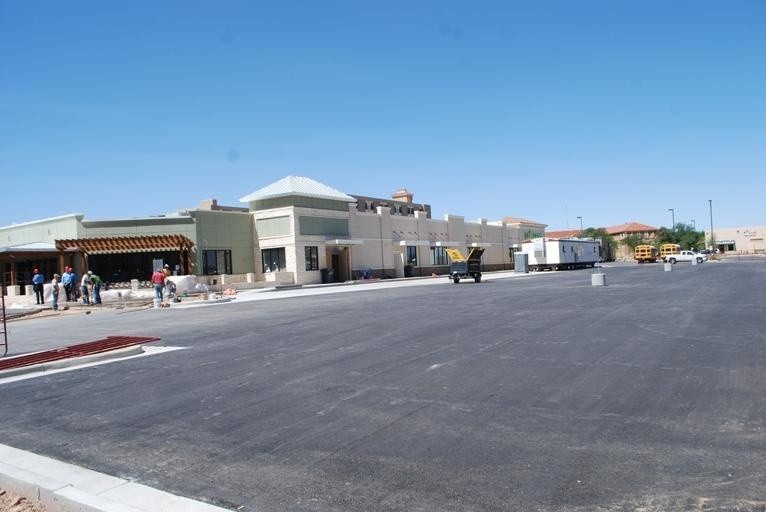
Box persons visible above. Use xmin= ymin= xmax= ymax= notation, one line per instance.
xmin=81 ymin=270 xmax=93 ymax=304
xmin=32 ymin=269 xmax=44 ymax=305
xmin=152 ymin=269 xmax=164 ymax=302
xmin=67 ymin=268 xmax=78 ymax=302
xmin=88 ymin=270 xmax=103 ymax=304
xmin=162 ymin=264 xmax=170 ymax=280
xmin=61 ymin=266 xmax=72 ymax=302
xmin=166 ymin=280 xmax=176 ymax=298
xmin=52 ymin=274 xmax=61 ymax=311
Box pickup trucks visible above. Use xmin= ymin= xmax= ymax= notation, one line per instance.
xmin=664 ymin=250 xmax=708 ymax=264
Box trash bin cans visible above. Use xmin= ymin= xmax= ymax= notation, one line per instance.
xmin=320 ymin=268 xmax=336 ymax=284
xmin=404 ymin=263 xmax=415 ymax=278
